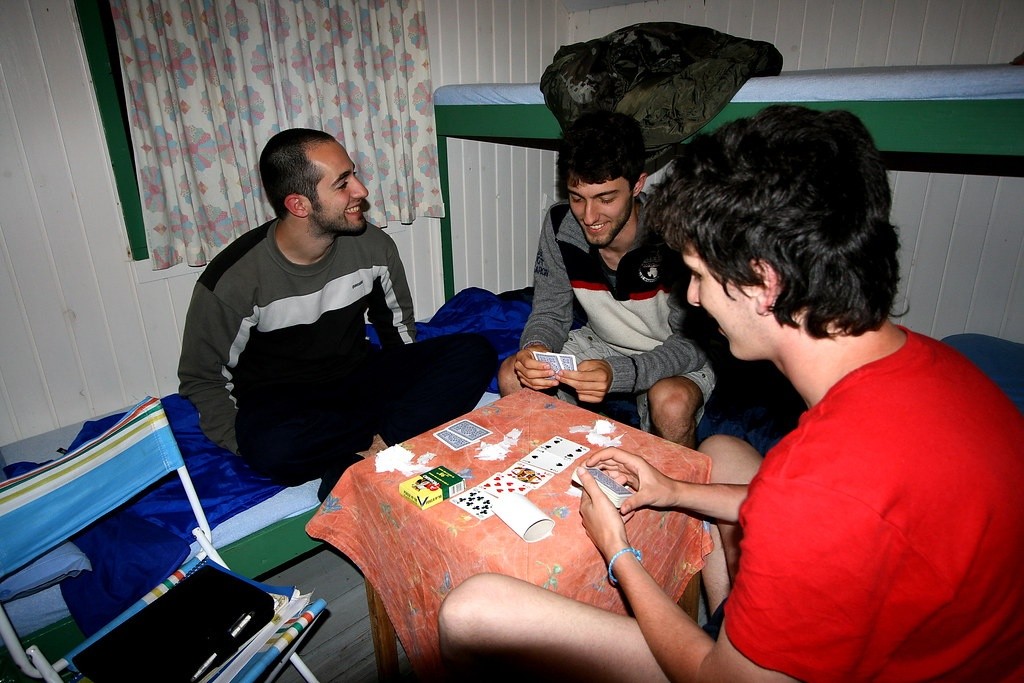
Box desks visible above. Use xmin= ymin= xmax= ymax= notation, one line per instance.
xmin=305 ymin=388 xmax=715 ymax=683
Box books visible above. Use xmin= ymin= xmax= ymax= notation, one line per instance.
xmin=71 ymin=552 xmax=300 ymax=683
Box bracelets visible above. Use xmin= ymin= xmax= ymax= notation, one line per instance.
xmin=607 ymin=548 xmax=642 ymax=583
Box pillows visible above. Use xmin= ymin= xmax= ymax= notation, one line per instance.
xmin=940 ymin=333 xmax=1024 ymax=416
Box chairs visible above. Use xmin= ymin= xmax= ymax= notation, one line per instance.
xmin=0 ymin=396 xmax=327 ymax=683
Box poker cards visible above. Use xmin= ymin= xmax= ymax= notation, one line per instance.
xmin=566 ymin=465 xmax=635 ymax=509
xmin=448 ymin=435 xmax=590 ymax=521
xmin=533 ymin=349 xmax=578 ymax=379
xmin=433 ymin=418 xmax=494 ymax=452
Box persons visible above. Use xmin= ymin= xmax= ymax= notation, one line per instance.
xmin=436 ymin=103 xmax=1024 ymax=682
xmin=496 ymin=106 xmax=721 ymax=454
xmin=179 ymin=128 xmax=498 ymax=506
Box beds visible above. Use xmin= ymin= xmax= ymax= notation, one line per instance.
xmin=0 ymin=315 xmax=500 ymax=683
xmin=433 ymin=64 xmax=1024 ymax=459
